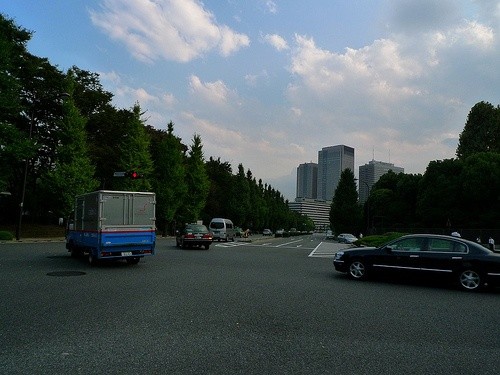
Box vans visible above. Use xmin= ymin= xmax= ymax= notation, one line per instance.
xmin=210 ymin=218 xmax=237 ymax=243
xmin=326 ymin=230 xmax=335 ymax=239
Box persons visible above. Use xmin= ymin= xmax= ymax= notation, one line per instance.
xmin=488 ymin=235 xmax=495 ymax=253
xmin=360 ymin=232 xmax=363 ymax=238
xmin=476 ymin=236 xmax=482 ymax=245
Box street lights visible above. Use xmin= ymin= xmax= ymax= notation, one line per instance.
xmin=16 ymin=91 xmax=71 ymax=240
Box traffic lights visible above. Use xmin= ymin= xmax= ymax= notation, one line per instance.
xmin=128 ymin=170 xmax=145 ymax=179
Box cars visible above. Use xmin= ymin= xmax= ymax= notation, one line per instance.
xmin=175 ymin=223 xmax=213 ymax=250
xmin=263 ymin=229 xmax=273 ymax=236
xmin=332 ymin=233 xmax=500 ymax=293
xmin=276 ymin=228 xmax=314 ymax=234
xmin=337 ymin=234 xmax=358 ymax=244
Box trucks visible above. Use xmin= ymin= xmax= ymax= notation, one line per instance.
xmin=64 ymin=191 xmax=157 ymax=267
xmin=290 ymin=228 xmax=296 ymax=233
